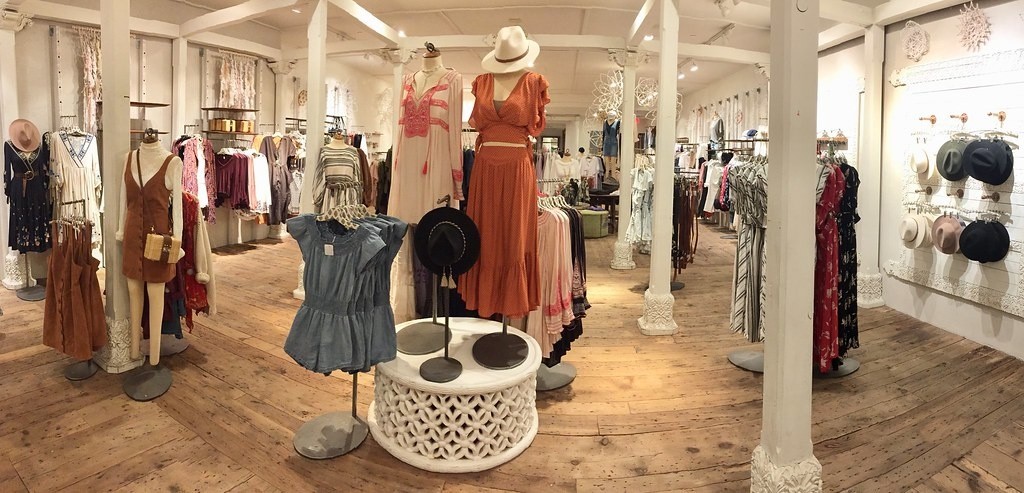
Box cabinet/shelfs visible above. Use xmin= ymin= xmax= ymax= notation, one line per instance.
xmin=202 ymin=107 xmax=260 ymax=142
xmin=95 ymin=99 xmax=170 ymax=136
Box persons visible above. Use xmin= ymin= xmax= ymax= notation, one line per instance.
xmin=600 ymin=110 xmax=622 ymax=180
xmin=3 ymin=119 xmax=53 ymax=301
xmin=310 ymin=131 xmax=366 ymax=218
xmin=115 ymin=127 xmax=184 ymax=367
xmin=450 ymin=24 xmax=550 ymax=372
xmin=705 ymin=110 xmax=725 ymax=145
xmin=385 ymin=41 xmax=465 ymax=357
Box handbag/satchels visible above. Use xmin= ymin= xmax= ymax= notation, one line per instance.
xmin=144 ymin=233 xmax=181 ymax=265
xmin=210 ymin=117 xmax=255 ymax=133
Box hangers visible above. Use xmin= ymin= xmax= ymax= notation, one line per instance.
xmin=4 ymin=116 xmax=1020 ymax=249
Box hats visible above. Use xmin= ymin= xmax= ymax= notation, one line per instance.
xmin=896 ymin=213 xmax=1010 ymax=264
xmin=963 ymin=138 xmax=1014 ymax=190
xmin=481 ymin=25 xmax=541 ymax=74
xmin=412 ymin=207 xmax=481 ymax=288
xmin=905 ymin=143 xmax=934 ymax=181
xmin=8 ymin=118 xmax=41 ymax=152
xmin=936 ymin=139 xmax=968 ymax=181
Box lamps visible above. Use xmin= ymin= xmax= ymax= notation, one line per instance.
xmin=733 ymin=0 xmax=741 ymax=6
xmin=716 ymin=0 xmax=731 ymax=18
xmin=593 ymin=30 xmax=698 ymax=117
